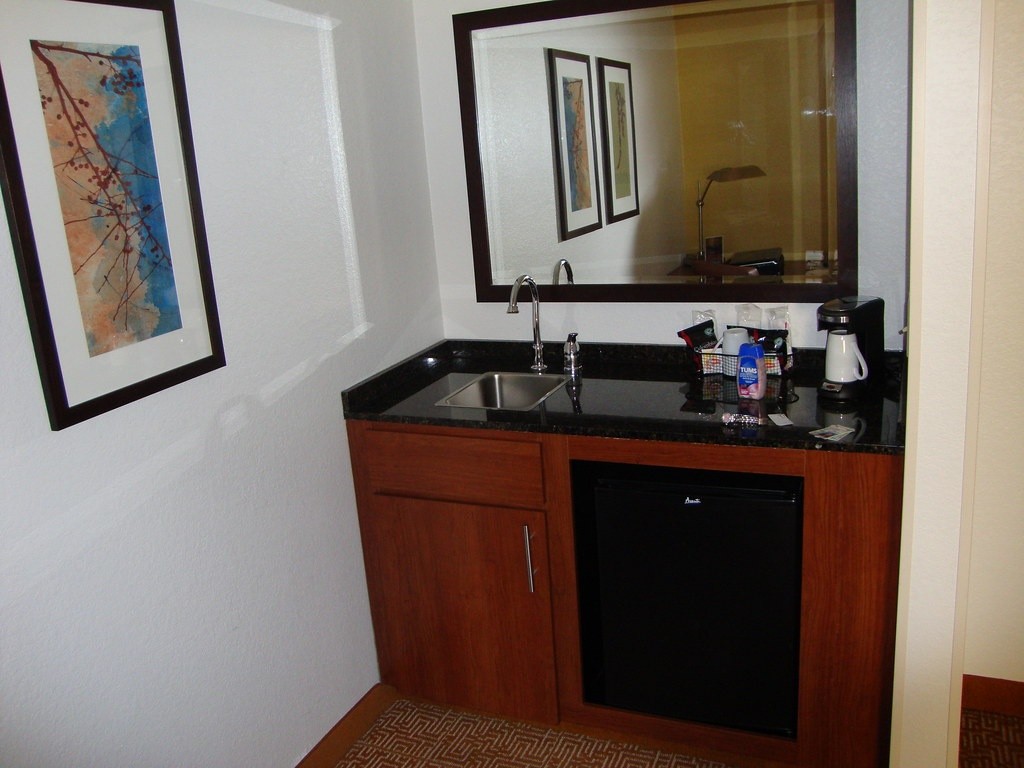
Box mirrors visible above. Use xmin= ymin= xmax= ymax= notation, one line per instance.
xmin=450 ymin=0 xmax=859 ymax=304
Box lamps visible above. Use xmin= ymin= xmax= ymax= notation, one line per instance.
xmin=696 ymin=165 xmax=767 ymax=259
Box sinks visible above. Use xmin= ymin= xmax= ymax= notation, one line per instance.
xmin=435 ymin=373 xmax=575 ymax=410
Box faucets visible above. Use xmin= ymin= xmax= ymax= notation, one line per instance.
xmin=507 ymin=275 xmax=547 ymax=370
xmin=553 ymin=259 xmax=574 ymax=285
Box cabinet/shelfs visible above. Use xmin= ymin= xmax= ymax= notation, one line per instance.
xmin=344 ymin=420 xmax=580 ymax=729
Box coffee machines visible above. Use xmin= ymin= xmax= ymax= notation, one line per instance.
xmin=816 ymin=295 xmax=885 ymax=444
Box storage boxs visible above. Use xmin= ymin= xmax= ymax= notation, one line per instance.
xmin=686 ymin=375 xmax=801 ymax=406
xmin=728 ymin=247 xmax=785 ymax=277
xmin=688 ymin=350 xmax=799 ymax=376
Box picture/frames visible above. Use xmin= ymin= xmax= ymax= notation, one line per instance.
xmin=596 ymin=56 xmax=641 ymax=228
xmin=547 ymin=48 xmax=603 ymax=244
xmin=0 ymin=0 xmax=227 ymax=434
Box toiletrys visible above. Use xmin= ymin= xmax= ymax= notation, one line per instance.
xmin=735 ymin=342 xmax=767 ymax=400
xmin=562 ymin=333 xmax=583 ymax=374
